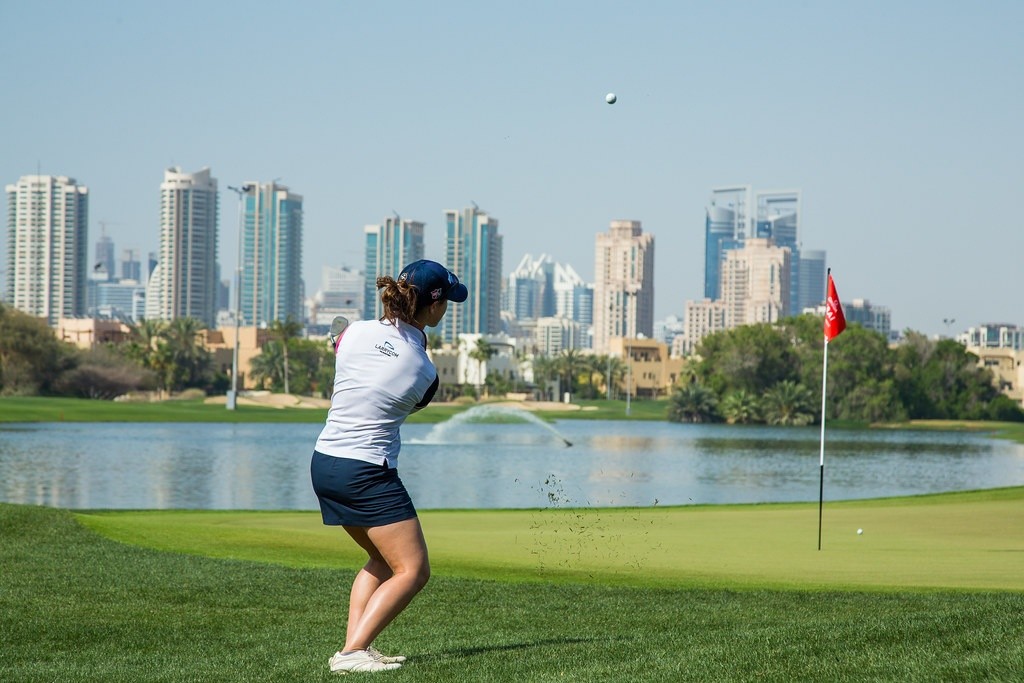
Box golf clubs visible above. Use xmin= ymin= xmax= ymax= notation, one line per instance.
xmin=330 ymin=316 xmax=348 ymax=347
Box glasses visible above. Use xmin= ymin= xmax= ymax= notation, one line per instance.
xmin=439 ymin=268 xmax=459 ymax=304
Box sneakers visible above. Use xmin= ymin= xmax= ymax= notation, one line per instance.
xmin=328 ymin=645 xmax=407 ymax=666
xmin=330 ymin=651 xmax=402 ymax=675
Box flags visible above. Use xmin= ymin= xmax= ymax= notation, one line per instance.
xmin=824 ymin=275 xmax=847 ymax=343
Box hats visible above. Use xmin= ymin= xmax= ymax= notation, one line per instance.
xmin=397 ymin=259 xmax=468 ymax=304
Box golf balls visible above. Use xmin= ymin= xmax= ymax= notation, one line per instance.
xmin=606 ymin=93 xmax=617 ymax=104
xmin=857 ymin=528 xmax=863 ymax=535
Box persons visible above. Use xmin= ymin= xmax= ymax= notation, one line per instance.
xmin=311 ymin=260 xmax=468 ymax=672
